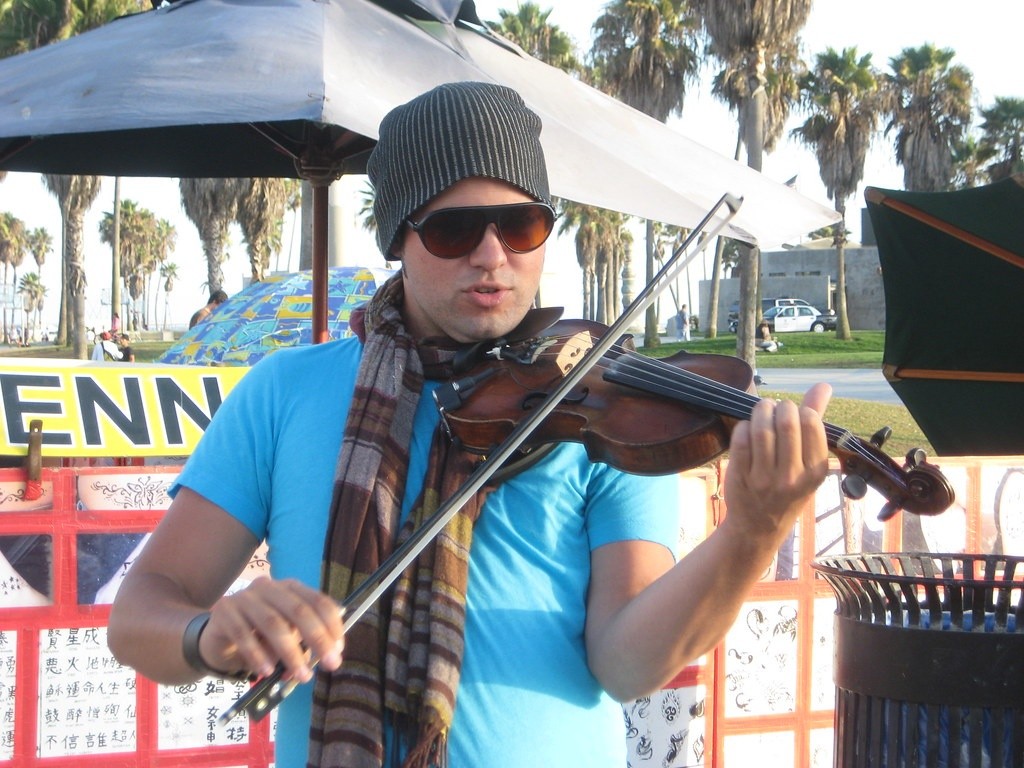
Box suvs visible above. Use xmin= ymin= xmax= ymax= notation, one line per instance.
xmin=728 ymin=298 xmax=817 ymax=325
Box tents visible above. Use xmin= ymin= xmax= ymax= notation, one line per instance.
xmin=153 ymin=264 xmax=401 ymax=371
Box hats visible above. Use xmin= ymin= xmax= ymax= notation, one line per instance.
xmin=366 ymin=83 xmax=557 ymax=261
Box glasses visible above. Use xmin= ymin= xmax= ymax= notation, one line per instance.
xmin=405 ymin=202 xmax=556 ymax=259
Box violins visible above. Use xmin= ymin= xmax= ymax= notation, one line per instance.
xmin=441 ymin=316 xmax=958 ymax=525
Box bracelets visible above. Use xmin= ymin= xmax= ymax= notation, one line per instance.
xmin=181 ymin=611 xmax=258 ymax=682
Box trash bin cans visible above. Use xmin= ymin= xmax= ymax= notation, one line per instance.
xmin=809 ymin=551 xmax=1024 ymax=768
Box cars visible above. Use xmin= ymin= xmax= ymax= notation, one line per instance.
xmin=729 ymin=305 xmax=826 ymax=333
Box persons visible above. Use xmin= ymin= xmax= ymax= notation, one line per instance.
xmin=106 ymin=82 xmax=835 ymax=767
xmin=92 ymin=329 xmax=135 ymax=363
xmin=675 ymin=304 xmax=690 ymax=342
xmin=44 ymin=328 xmax=49 ymax=342
xmin=755 ymin=317 xmax=778 ymax=352
xmin=189 ymin=289 xmax=228 ymax=331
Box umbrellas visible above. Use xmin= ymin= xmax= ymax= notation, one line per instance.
xmin=371 ymin=0 xmax=844 ymax=253
xmin=0 ymin=0 xmax=759 ymax=350
